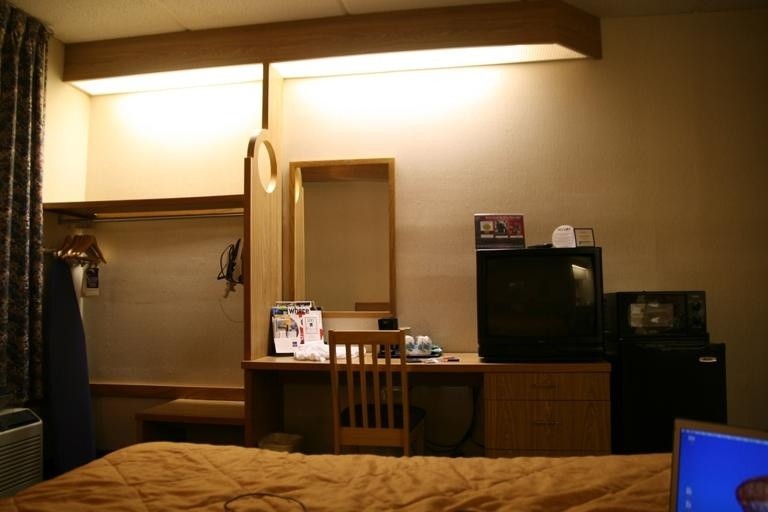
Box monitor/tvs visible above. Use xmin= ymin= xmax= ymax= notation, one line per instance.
xmin=476 ymin=246 xmax=603 ymax=364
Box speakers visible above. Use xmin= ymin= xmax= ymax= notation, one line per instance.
xmin=378 ymin=318 xmax=398 ymax=354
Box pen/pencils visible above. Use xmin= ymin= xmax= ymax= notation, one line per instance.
xmin=406 ymin=356 xmax=460 ymax=363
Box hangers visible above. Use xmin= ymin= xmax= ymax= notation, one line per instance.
xmin=54 ymin=219 xmax=109 ymax=266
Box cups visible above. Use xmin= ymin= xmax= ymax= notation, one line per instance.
xmin=405 ymin=335 xmax=433 ymax=355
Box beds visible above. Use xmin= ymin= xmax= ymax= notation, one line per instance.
xmin=0 ymin=439 xmax=672 ymax=512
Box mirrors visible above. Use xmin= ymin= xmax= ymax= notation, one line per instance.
xmin=288 ymin=158 xmax=397 ymax=319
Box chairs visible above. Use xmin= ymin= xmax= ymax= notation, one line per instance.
xmin=329 ymin=330 xmax=426 ymax=457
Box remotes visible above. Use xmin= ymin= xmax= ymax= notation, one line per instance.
xmin=527 ymin=243 xmax=552 ymax=247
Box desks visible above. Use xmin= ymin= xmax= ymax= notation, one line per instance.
xmin=242 ymin=352 xmax=613 ymax=456
xmin=134 ymin=397 xmax=246 ymax=444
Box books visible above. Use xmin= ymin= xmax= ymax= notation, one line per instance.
xmin=275 ymin=301 xmax=315 ymax=307
xmin=270 ymin=305 xmax=324 ymax=354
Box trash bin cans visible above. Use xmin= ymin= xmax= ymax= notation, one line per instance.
xmin=258 ymin=431 xmax=303 ymax=452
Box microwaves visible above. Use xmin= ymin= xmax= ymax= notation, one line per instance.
xmin=602 ymin=290 xmax=708 ymax=345
